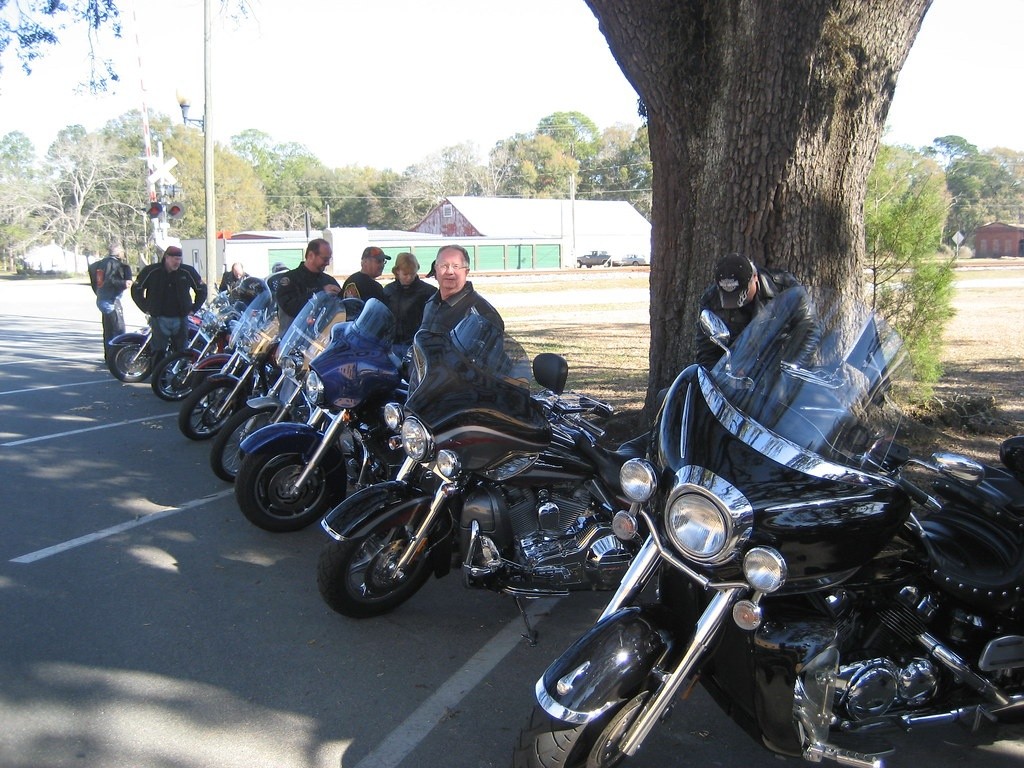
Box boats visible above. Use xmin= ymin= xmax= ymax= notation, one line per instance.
xmin=147 ymin=203 xmax=163 ymax=220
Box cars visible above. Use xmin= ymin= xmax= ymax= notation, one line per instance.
xmin=577 ymin=249 xmax=612 ymax=267
xmin=612 ymin=253 xmax=652 ymax=267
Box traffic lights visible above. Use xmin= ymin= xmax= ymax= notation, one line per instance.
xmin=170 ymin=204 xmax=184 ymax=218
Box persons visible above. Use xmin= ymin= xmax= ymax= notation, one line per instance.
xmin=131 ymin=246 xmax=207 ymax=390
xmin=425 ymin=259 xmax=438 ymax=282
xmin=410 ymin=245 xmax=505 ymax=383
xmin=693 ymin=254 xmax=821 ymax=399
xmin=218 ymin=263 xmax=251 ymax=294
xmin=271 ymin=262 xmax=290 ymax=274
xmin=338 ymin=247 xmax=391 ymax=304
xmin=276 ymin=239 xmax=341 ymax=319
xmin=380 ymin=252 xmax=439 ymax=346
xmin=89 ymin=243 xmax=132 ymax=365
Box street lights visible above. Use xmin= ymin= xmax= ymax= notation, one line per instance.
xmin=172 ymin=86 xmax=217 ymax=304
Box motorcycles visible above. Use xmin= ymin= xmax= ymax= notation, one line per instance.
xmin=107 ymin=274 xmax=659 ymax=641
xmin=511 ymin=283 xmax=1023 ymax=768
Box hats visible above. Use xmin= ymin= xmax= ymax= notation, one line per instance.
xmin=361 ymin=247 xmax=391 ymax=260
xmin=426 ymin=260 xmax=436 ymax=278
xmin=165 ymin=246 xmax=181 ymax=255
xmin=714 ymin=252 xmax=753 ymax=309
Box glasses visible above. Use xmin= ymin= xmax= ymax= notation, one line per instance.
xmin=433 ymin=263 xmax=468 ymax=272
xmin=315 ymin=254 xmax=333 ymax=262
xmin=371 ymin=258 xmax=388 ymax=265
